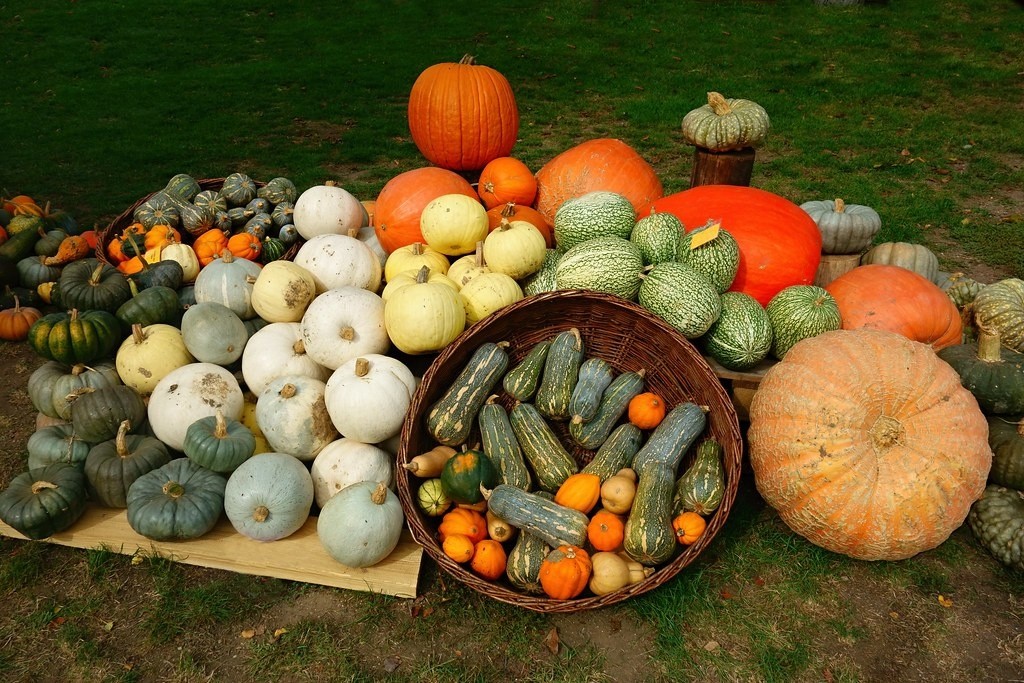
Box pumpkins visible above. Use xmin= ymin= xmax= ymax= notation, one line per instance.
xmin=682 ymin=92 xmax=770 ymax=152
xmin=0 ymin=133 xmax=1024 ymax=597
xmin=408 ymin=54 xmax=518 ymax=171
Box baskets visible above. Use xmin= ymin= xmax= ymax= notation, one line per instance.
xmin=397 ymin=288 xmax=741 ymax=612
